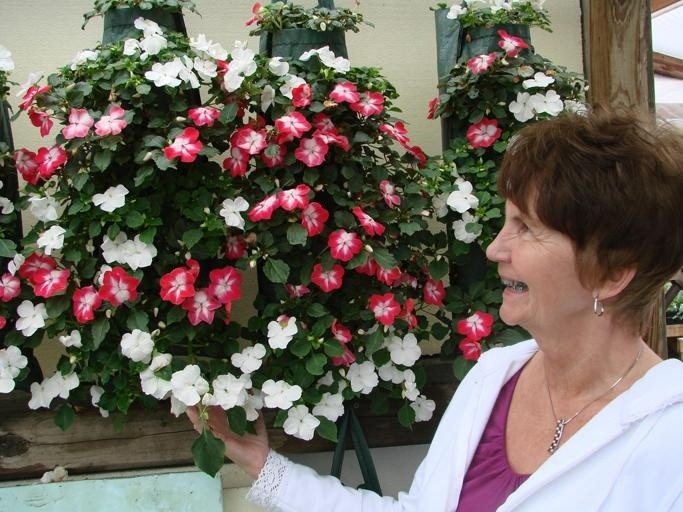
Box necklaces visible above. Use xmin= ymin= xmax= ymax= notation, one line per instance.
xmin=539 ymin=339 xmax=642 ymax=459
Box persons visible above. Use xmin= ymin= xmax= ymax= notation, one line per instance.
xmin=183 ymin=105 xmax=683 ymax=512
